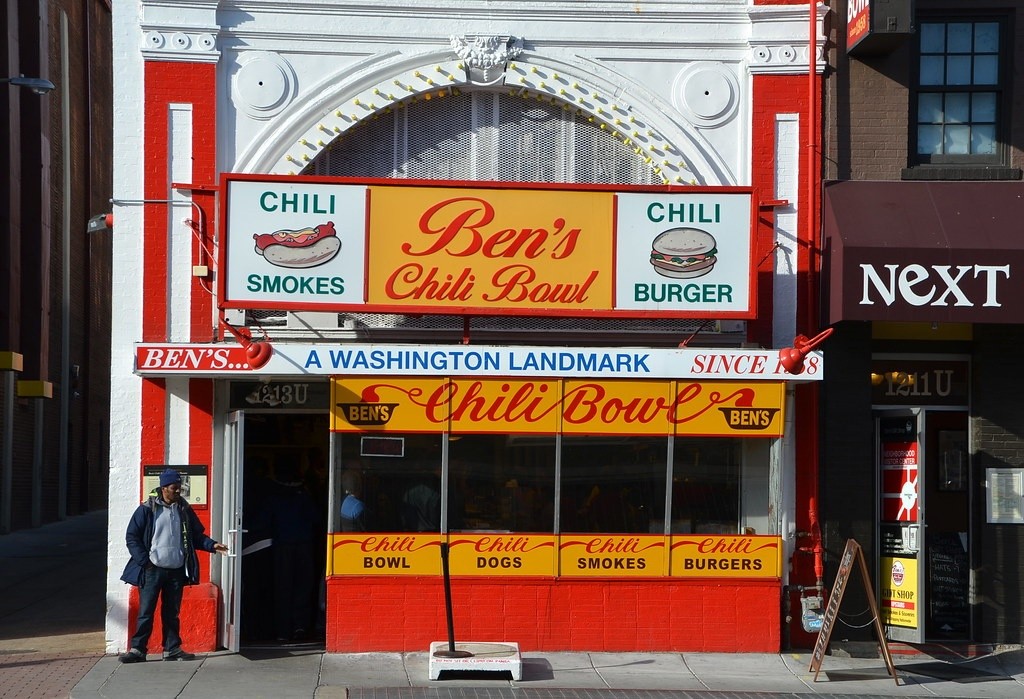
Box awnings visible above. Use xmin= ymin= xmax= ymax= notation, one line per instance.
xmin=820 ymin=183 xmax=1024 ymax=327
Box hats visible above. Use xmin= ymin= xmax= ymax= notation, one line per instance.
xmin=159 ymin=468 xmax=182 ymax=488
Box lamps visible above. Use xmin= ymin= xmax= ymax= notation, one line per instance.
xmin=87 ymin=213 xmax=113 ymax=233
xmin=932 ymin=321 xmax=939 ymax=330
xmin=778 ymin=326 xmax=834 ymax=375
xmin=219 ymin=316 xmax=273 ymax=370
xmin=871 ymin=371 xmax=915 ymax=386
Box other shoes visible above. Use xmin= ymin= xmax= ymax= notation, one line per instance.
xmin=118 ymin=651 xmax=146 ymax=663
xmin=163 ymin=650 xmax=195 ymax=661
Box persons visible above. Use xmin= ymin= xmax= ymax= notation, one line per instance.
xmin=121 ymin=470 xmax=227 ymax=663
xmin=245 ymin=446 xmax=327 ymax=641
xmin=342 ymin=471 xmax=391 ymax=532
xmin=400 ymin=448 xmax=723 ymax=534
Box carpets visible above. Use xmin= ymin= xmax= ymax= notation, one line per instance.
xmin=893 ymin=661 xmax=1014 ymax=683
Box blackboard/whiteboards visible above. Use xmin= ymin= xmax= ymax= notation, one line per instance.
xmin=808 ymin=539 xmax=858 ymax=671
xmin=926 ymin=529 xmax=970 ymax=635
